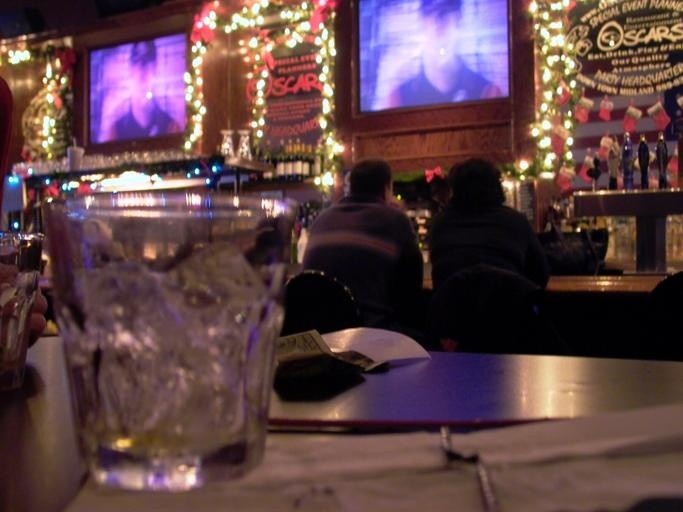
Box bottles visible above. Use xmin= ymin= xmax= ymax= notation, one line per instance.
xmin=275 ymin=137 xmax=314 ymax=182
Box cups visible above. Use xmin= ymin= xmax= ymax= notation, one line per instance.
xmin=43 ymin=190 xmax=300 ymax=495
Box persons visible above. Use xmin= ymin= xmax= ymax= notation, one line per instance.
xmin=0 ymin=263 xmax=48 ymax=349
xmin=104 ymin=40 xmax=181 ymax=141
xmin=431 ymin=159 xmax=549 ymax=292
xmin=302 ymin=159 xmax=423 ymax=327
xmin=385 ymin=0 xmax=504 ymax=109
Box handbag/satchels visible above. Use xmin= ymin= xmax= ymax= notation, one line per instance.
xmin=539 ymin=227 xmax=608 ymax=275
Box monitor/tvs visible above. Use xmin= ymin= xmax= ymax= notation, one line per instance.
xmin=358 ymin=0 xmax=510 ymax=115
xmin=88 ymin=32 xmax=186 ymax=144
